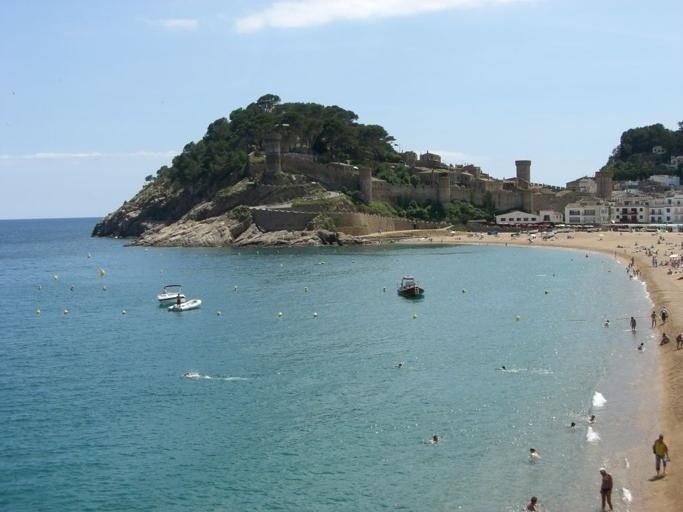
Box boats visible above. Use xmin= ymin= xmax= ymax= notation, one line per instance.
xmin=159 ymin=292 xmax=186 ymax=306
xmin=397 ymin=276 xmax=424 ymax=299
xmin=168 ymin=299 xmax=202 ymax=311
